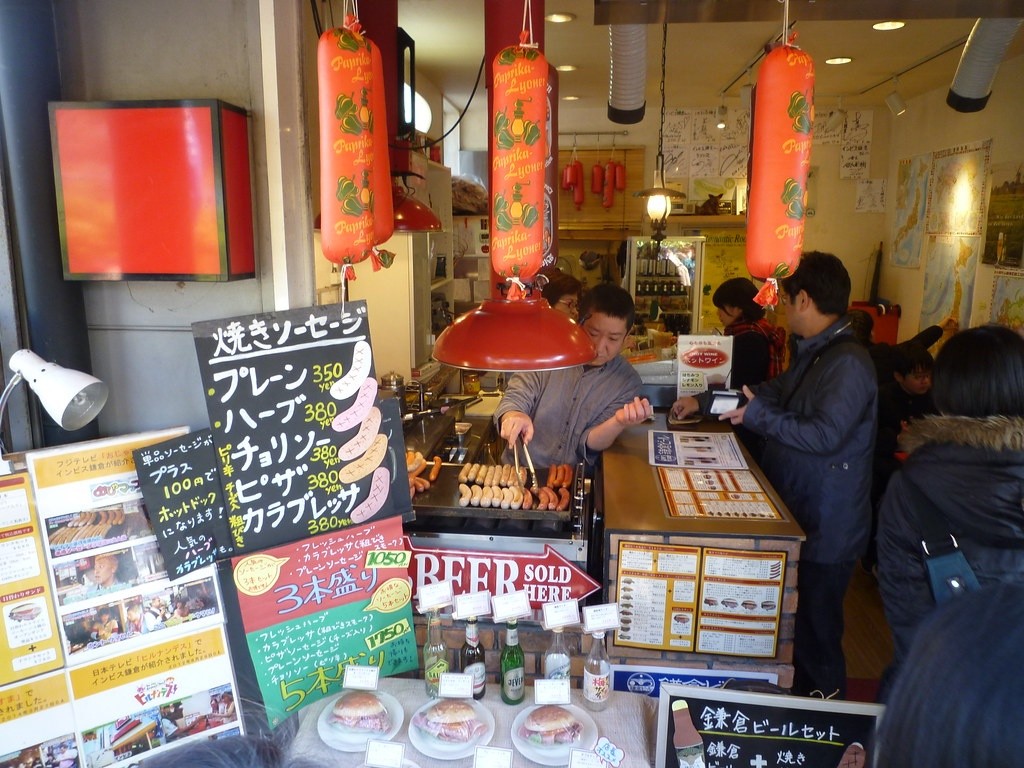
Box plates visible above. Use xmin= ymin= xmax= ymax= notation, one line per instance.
xmin=408 ymin=697 xmax=495 ymax=760
xmin=318 ymin=691 xmax=405 ymax=752
xmin=511 ymin=703 xmax=599 ymax=765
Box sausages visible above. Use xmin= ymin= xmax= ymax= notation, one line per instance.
xmin=458 ymin=462 xmax=527 ymax=509
xmin=522 ymin=463 xmax=574 ymax=511
xmin=48 ymin=508 xmax=124 ymax=545
xmin=405 ymin=450 xmax=442 ymax=500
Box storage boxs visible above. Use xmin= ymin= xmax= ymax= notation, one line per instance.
xmin=411 ymin=361 xmax=440 ymax=377
xmin=473 ymin=281 xmax=490 ymax=303
xmin=453 ymin=277 xmax=478 ymax=302
xmin=431 ymin=253 xmax=447 ymax=280
xmin=46 ymin=98 xmax=257 ymax=284
xmin=478 ymin=258 xmax=490 ymax=280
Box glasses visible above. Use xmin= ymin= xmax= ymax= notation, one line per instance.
xmin=557 ymin=298 xmax=581 ymax=312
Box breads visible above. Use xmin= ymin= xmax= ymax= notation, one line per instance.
xmin=524 ymin=705 xmax=575 ymax=730
xmin=428 ymin=700 xmax=476 ymax=723
xmin=332 ymin=691 xmax=384 ymax=716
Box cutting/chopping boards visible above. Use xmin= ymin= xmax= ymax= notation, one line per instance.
xmin=438 ymin=393 xmax=502 ymax=417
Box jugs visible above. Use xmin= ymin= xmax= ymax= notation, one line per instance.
xmin=381 ymin=370 xmax=420 ymax=418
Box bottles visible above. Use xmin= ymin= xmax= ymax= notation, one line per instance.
xmin=636 ymin=281 xmax=687 ymax=311
xmin=461 ymin=614 xmax=485 ymax=698
xmin=499 ymin=619 xmax=525 ymax=704
xmin=544 ymin=626 xmax=570 ymax=680
xmin=423 ymin=609 xmax=450 ymax=697
xmin=636 ymin=247 xmax=694 ymax=276
xmin=634 ymin=313 xmax=689 ymax=337
xmin=584 ymin=630 xmax=610 ymax=711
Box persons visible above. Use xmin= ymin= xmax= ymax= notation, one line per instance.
xmin=534 ymin=267 xmax=579 ymax=322
xmin=840 ymin=303 xmax=961 ymax=576
xmin=494 ymin=285 xmax=650 ymax=470
xmin=161 ymin=691 xmax=237 ymax=745
xmin=64 ymin=553 xmax=217 ymax=656
xmin=670 ymin=248 xmax=878 ymax=699
xmin=876 ymin=321 xmax=1024 ymax=705
xmin=875 ymin=577 xmax=1024 ymax=768
xmin=9 ymin=743 xmax=78 ymax=768
xmin=705 ymin=275 xmax=773 ymax=399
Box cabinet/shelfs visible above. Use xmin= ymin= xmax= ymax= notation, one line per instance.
xmin=313 ymin=158 xmax=456 ymax=386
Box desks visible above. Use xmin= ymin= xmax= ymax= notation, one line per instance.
xmin=600 ymin=412 xmax=808 ymax=689
xmin=285 ymin=674 xmax=658 ymax=768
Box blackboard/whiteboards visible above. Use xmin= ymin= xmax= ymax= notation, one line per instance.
xmin=190 ymin=297 xmax=414 ymax=557
xmin=654 ymin=682 xmax=886 ymax=768
xmin=131 ymin=427 xmax=235 ymax=582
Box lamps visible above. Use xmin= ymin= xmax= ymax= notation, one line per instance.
xmin=824 ymin=93 xmax=847 ymax=132
xmin=0 ymin=347 xmax=111 ymax=453
xmin=716 ymin=92 xmax=728 ymax=129
xmin=877 ymin=71 xmax=910 ymax=117
xmin=631 ymin=23 xmax=688 ymax=254
xmin=740 ymin=67 xmax=754 ymax=108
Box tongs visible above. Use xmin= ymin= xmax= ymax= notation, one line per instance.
xmin=512 ymin=429 xmax=537 ymax=489
